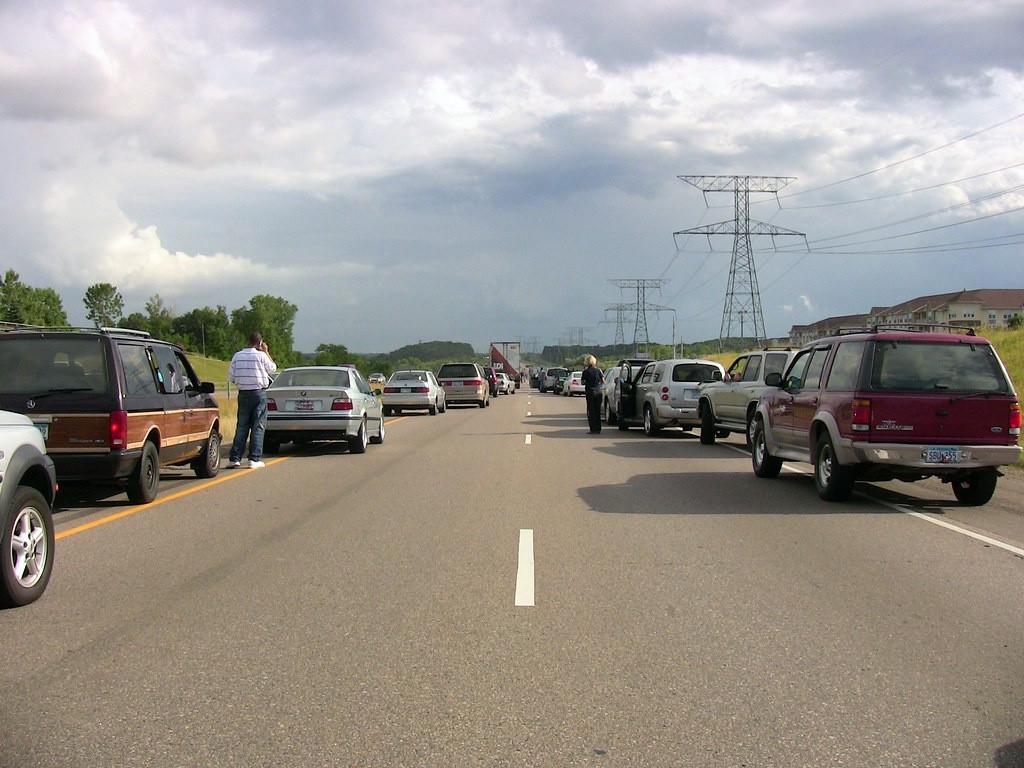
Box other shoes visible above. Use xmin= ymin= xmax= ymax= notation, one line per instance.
xmin=586 ymin=431 xmax=600 ymax=434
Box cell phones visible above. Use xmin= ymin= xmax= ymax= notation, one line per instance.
xmin=259 ymin=341 xmax=263 ymax=346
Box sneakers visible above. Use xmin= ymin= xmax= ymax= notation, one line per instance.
xmin=248 ymin=460 xmax=265 ymax=468
xmin=225 ymin=461 xmax=241 ymax=469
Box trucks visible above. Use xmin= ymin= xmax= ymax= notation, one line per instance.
xmin=487 ymin=341 xmax=523 ymax=389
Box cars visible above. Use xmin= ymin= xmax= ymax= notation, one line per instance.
xmin=369 ymin=372 xmax=385 ymax=384
xmin=525 ymin=366 xmax=544 ymax=388
xmin=563 ymin=371 xmax=587 ymax=397
xmin=382 ymin=370 xmax=446 ymax=417
xmin=0 ymin=410 xmax=59 ymax=607
xmin=262 ymin=366 xmax=385 ymax=455
xmin=496 ymin=372 xmax=515 ymax=395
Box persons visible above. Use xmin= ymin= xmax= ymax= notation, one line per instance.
xmin=223 ymin=330 xmax=277 ymax=468
xmin=520 ymin=369 xmax=527 ymax=383
xmin=733 ymin=372 xmax=741 ymax=382
xmin=580 ymin=355 xmax=605 ymax=434
xmin=538 ymin=367 xmax=547 ymax=392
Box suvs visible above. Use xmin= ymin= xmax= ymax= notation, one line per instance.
xmin=435 ymin=362 xmax=491 ymax=408
xmin=539 ymin=367 xmax=569 ymax=393
xmin=752 ymin=322 xmax=1023 ymax=508
xmin=697 ymin=345 xmax=808 ymax=453
xmin=614 ymin=358 xmax=733 ymax=438
xmin=588 ymin=366 xmax=648 ymax=426
xmin=552 ymin=369 xmax=573 ymax=395
xmin=482 ymin=366 xmax=500 ymax=397
xmin=0 ymin=322 xmax=223 ymax=505
